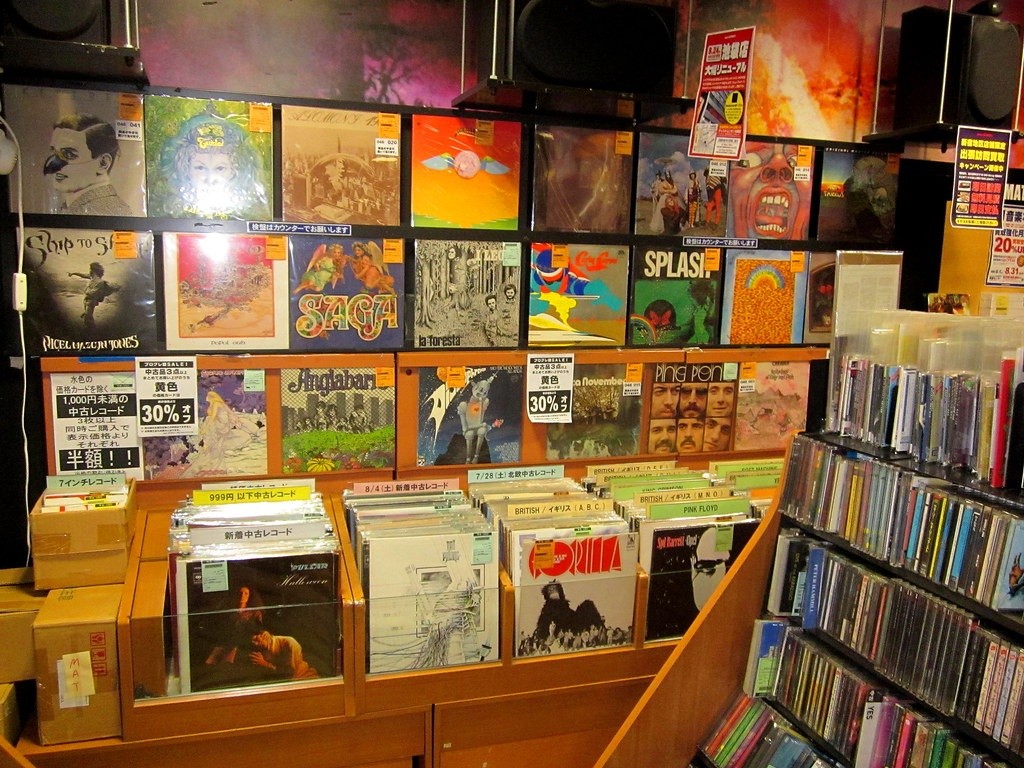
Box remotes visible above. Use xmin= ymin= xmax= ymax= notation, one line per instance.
xmin=967 ymin=0 xmax=1005 ymax=17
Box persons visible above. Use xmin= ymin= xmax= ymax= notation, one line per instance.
xmin=648 ymin=418 xmax=676 ymax=455
xmin=652 ymin=169 xmax=678 ymax=232
xmin=678 ymin=382 xmax=708 ymax=418
xmin=702 ymin=167 xmax=723 ymax=226
xmin=684 ymin=171 xmax=703 ymax=229
xmin=215 ymin=584 xmax=262 ymax=686
xmin=660 ymin=197 xmax=686 ymax=237
xmin=650 ymin=170 xmax=664 ymax=213
xmin=650 ymin=383 xmax=681 ymax=419
xmin=244 ymin=623 xmax=319 ymax=683
xmin=706 ymin=382 xmax=734 ymax=417
xmin=676 ymin=419 xmax=704 ymax=453
xmin=703 ymin=417 xmax=732 ymax=452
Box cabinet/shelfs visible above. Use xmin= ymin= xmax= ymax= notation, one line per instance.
xmin=0 ymin=341 xmax=1024 ymax=767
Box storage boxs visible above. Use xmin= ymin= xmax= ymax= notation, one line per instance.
xmin=31 ymin=583 xmax=123 ymax=744
xmin=27 ymin=476 xmax=137 ymax=592
xmin=0 ymin=681 xmax=20 ymax=744
xmin=0 ymin=566 xmax=37 ymax=683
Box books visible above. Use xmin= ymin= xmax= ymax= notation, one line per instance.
xmin=0 ymin=83 xmax=1024 ymax=768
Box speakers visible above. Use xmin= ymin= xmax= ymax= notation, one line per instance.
xmin=0 ymin=0 xmax=143 ymax=81
xmin=892 ymin=5 xmax=1024 ymax=131
xmin=477 ymin=0 xmax=678 ymax=96
